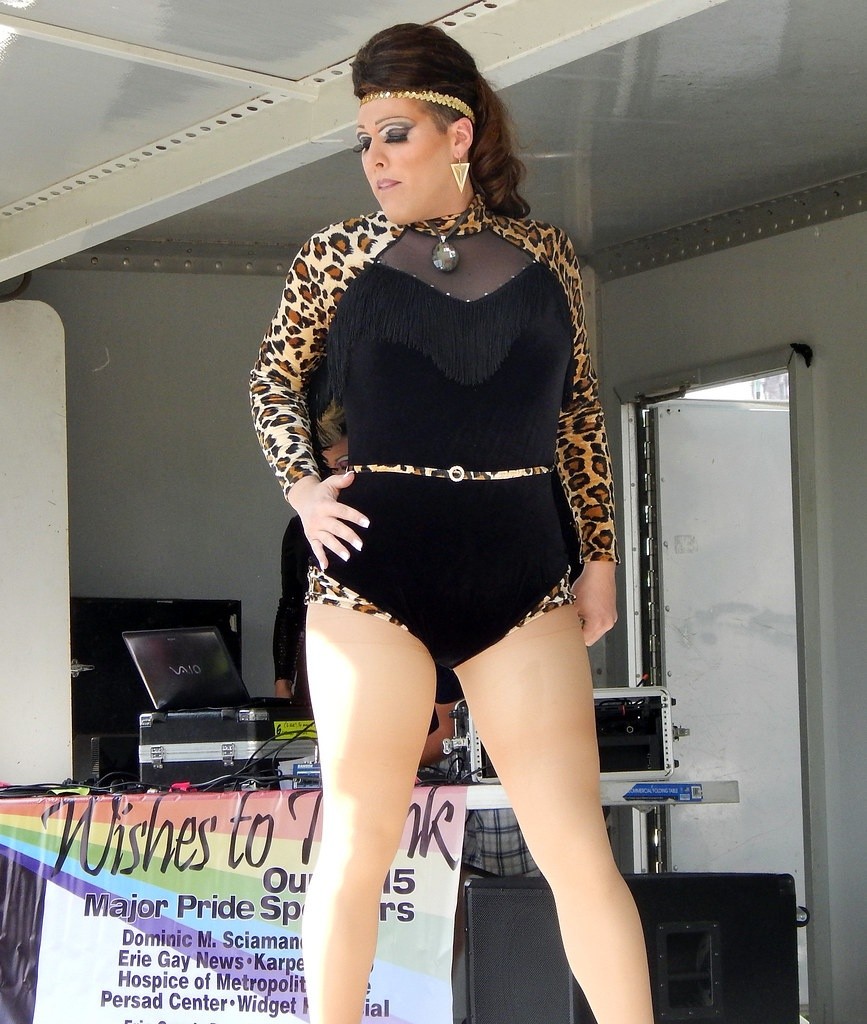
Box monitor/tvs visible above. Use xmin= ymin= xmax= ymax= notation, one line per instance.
xmin=69 ymin=597 xmax=242 ymax=733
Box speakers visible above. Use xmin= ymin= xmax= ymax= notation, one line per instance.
xmin=465 ymin=870 xmax=800 ymax=1024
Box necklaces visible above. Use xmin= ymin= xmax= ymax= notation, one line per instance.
xmin=425 ymin=208 xmax=472 ymax=273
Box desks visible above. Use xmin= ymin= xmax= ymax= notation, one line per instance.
xmin=0 ymin=781 xmax=747 ymax=1024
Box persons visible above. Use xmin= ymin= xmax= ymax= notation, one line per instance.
xmin=250 ymin=23 xmax=656 ymax=1024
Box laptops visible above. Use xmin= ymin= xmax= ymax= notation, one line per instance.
xmin=121 ymin=627 xmax=302 ymax=712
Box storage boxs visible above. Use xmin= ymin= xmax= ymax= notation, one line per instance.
xmin=137 ymin=704 xmax=318 ymax=785
